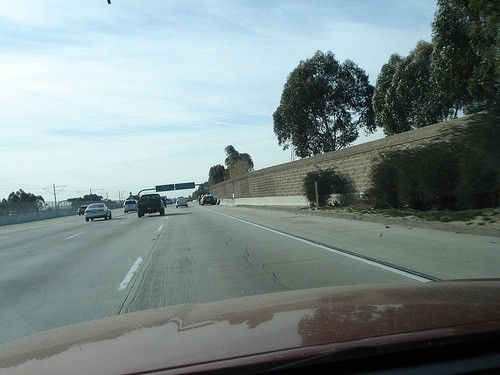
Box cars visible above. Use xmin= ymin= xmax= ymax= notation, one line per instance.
xmin=200 ymin=195 xmax=216 ymax=206
xmin=84 ymin=203 xmax=111 ymax=221
xmin=78 ymin=205 xmax=88 ymax=215
xmin=123 ymin=199 xmax=138 ymax=212
xmin=166 ymin=196 xmax=190 ymax=208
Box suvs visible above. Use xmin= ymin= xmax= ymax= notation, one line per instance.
xmin=137 ymin=194 xmax=165 ymax=217
